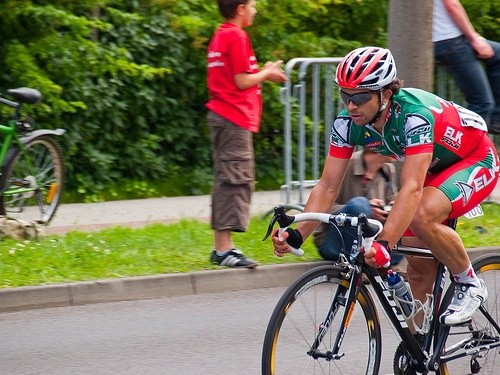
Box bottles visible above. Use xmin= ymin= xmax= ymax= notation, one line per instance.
xmin=387 ymin=270 xmax=417 ymax=320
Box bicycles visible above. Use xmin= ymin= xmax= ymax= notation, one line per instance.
xmin=1 ymin=82 xmax=70 ymax=234
xmin=260 ymin=204 xmax=500 ymax=375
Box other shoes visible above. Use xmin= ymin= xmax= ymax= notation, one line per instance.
xmin=391 ymin=264 xmax=409 ymax=281
xmin=362 ymin=273 xmax=370 ymax=285
xmin=488 ymin=120 xmax=500 ymax=135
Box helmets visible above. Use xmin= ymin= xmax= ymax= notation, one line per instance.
xmin=334 ymin=46 xmax=397 ymax=90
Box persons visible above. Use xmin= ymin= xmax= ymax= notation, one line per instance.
xmin=270 ymin=46 xmax=500 ymax=351
xmin=432 ymin=0 xmax=500 ymax=130
xmin=204 ymin=0 xmax=289 ymax=268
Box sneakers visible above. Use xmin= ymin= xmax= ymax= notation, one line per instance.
xmin=211 ymin=250 xmax=258 ymax=268
xmin=400 ymin=350 xmax=429 ymax=371
xmin=439 ymin=279 xmax=489 ymax=325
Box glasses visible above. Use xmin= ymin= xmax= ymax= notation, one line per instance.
xmin=340 ymin=90 xmax=377 ymax=105
